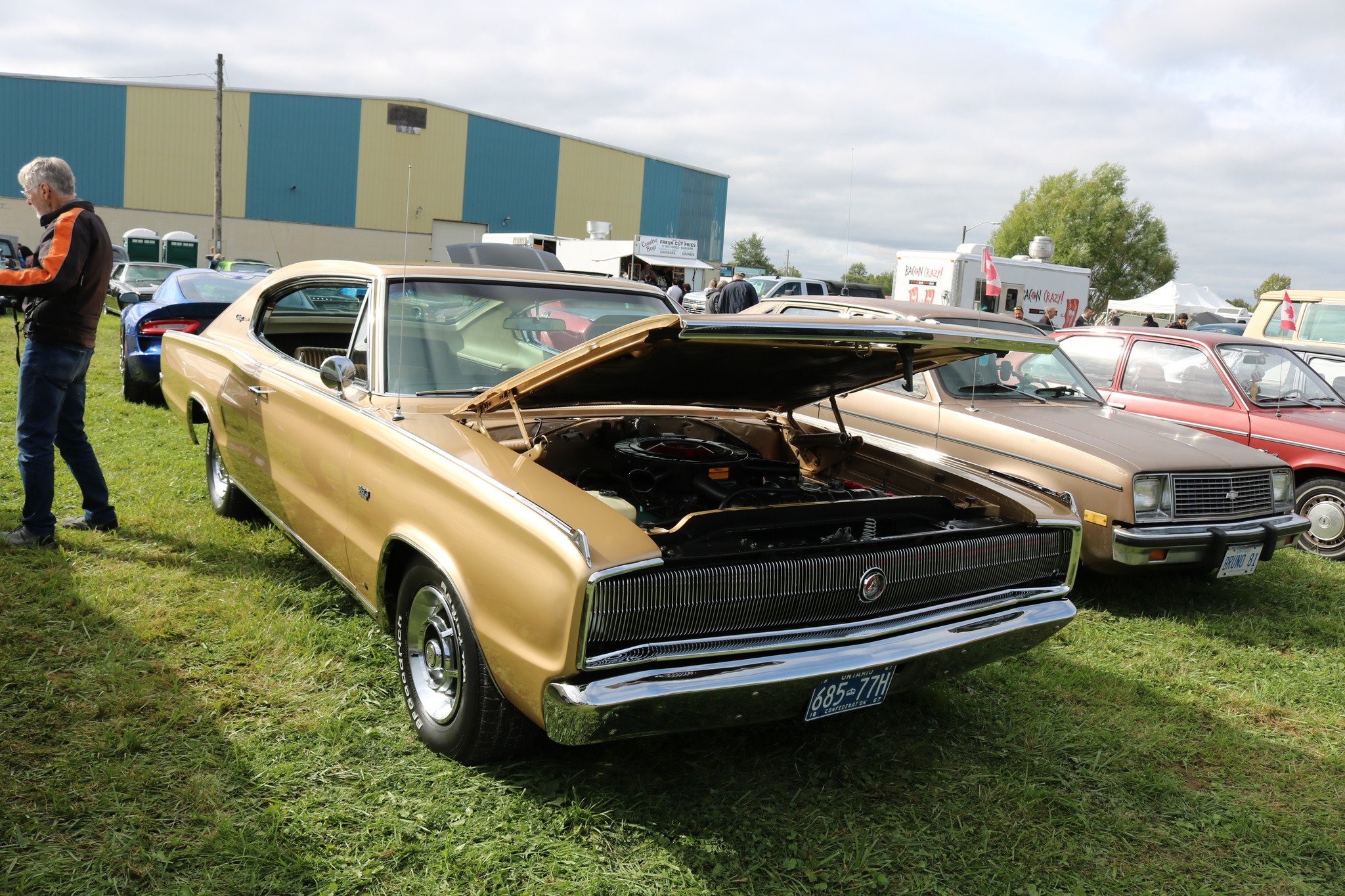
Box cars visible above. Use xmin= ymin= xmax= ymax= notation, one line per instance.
xmin=102 ymin=241 xmax=1083 ymax=771
xmin=0 ymin=235 xmax=22 ymax=272
xmin=993 ymin=322 xmax=1345 ymax=560
xmin=734 ymin=295 xmax=1311 ymax=578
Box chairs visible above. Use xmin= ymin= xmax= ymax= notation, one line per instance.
xmin=1132 ymin=363 xmax=1222 ymax=404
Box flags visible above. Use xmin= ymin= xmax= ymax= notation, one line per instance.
xmin=1279 ymin=292 xmax=1298 ymax=332
xmin=982 ymin=248 xmax=1001 ymax=297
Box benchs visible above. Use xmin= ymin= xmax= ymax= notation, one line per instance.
xmin=293 ymin=347 xmax=368 ymax=368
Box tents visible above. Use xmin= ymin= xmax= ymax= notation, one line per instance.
xmin=1105 ymin=280 xmax=1254 ymax=331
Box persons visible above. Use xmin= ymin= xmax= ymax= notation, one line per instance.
xmin=207 ymin=246 xmax=223 ymax=269
xmin=0 ymin=156 xmax=120 ymax=547
xmin=1106 ymin=309 xmax=1120 ymax=326
xmin=639 ymin=263 xmax=767 ymax=315
xmin=1014 ymin=306 xmax=1031 ymax=321
xmin=1075 ymin=306 xmax=1095 ymax=327
xmin=18 ymin=243 xmax=34 ymax=268
xmin=1038 ymin=306 xmax=1056 ymax=326
xmin=1141 ymin=314 xmax=1159 ymax=327
xmin=1165 ymin=313 xmax=1188 ymax=329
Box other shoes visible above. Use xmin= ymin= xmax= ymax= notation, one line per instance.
xmin=1 ymin=524 xmax=55 ymax=549
xmin=61 ymin=515 xmax=120 ymax=531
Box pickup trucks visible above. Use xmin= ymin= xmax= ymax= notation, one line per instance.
xmin=682 ymin=274 xmax=830 ymax=316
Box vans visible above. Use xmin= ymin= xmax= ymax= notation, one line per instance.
xmin=1224 ymin=290 xmax=1345 ymax=400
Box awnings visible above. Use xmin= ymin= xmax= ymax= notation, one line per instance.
xmin=634 ymin=253 xmax=714 ymax=269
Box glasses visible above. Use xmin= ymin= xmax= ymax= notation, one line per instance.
xmin=1013 ymin=307 xmax=1021 ymax=310
xmin=21 ymin=184 xmax=52 ymax=199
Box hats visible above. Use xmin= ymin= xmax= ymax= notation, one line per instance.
xmin=1178 ymin=313 xmax=1188 ymax=319
xmin=645 ymin=274 xmax=651 ymax=278
xmin=733 ymin=273 xmax=743 ymax=278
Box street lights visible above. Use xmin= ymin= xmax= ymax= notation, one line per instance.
xmin=961 ymin=221 xmax=1002 ymax=243
